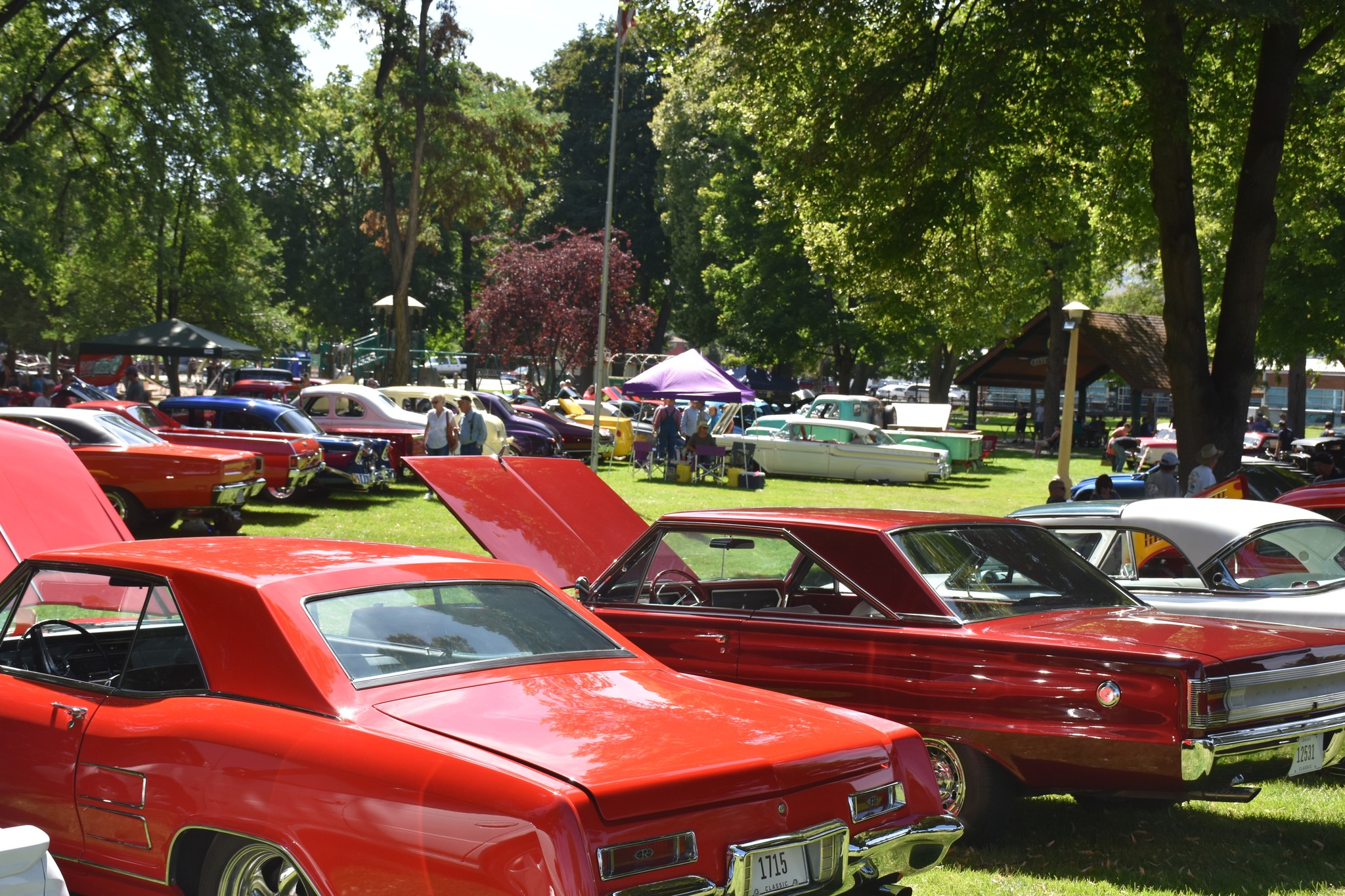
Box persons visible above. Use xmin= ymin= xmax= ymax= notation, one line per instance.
xmin=0 ymin=356 xmax=76 ymax=408
xmin=1274 ymin=420 xmax=1297 ymax=456
xmin=1245 ymin=411 xmax=1286 ymax=433
xmin=1011 ymin=402 xmax=1027 ymax=444
xmin=1046 ymin=481 xmax=1066 ymax=505
xmin=1310 ymin=451 xmax=1345 ymax=484
xmin=508 ymin=381 xmax=541 ymax=404
xmin=1090 ymin=475 xmax=1121 ymax=501
xmin=1184 ymin=444 xmax=1225 ymax=498
xmin=422 ymin=395 xmax=459 ymax=500
xmin=1320 ymin=421 xmax=1335 ymax=437
xmin=555 ymin=378 xmax=612 ymax=402
xmin=361 ymin=378 xmax=380 ymax=389
xmin=1144 ymin=452 xmax=1180 ymax=500
xmin=454 ymin=395 xmax=488 ymax=456
xmin=187 ymin=357 xmax=220 ymax=388
xmin=122 ymin=365 xmax=146 ymax=404
xmin=652 ymin=396 xmax=734 ymax=483
xmin=301 ymin=373 xmax=310 ymax=388
xmin=1032 ymin=396 xmax=1175 ymax=472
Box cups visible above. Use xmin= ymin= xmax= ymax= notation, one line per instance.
xmin=682 ymin=451 xmax=685 ymax=461
xmin=653 ymin=431 xmax=657 ymax=438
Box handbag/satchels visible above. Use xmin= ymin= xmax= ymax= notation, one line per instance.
xmin=445 ymin=409 xmax=456 ymax=448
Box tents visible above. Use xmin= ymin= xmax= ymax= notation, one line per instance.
xmin=78 ymin=316 xmax=264 ymax=397
xmin=725 ymin=364 xmax=800 ymax=398
xmin=608 ymin=348 xmax=760 ymax=490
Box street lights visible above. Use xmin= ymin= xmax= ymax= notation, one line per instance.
xmin=1061 ymin=300 xmax=1092 ymax=501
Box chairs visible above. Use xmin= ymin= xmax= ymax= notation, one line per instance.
xmin=632 ymin=436 xmax=757 ymax=486
xmin=982 ymin=435 xmax=997 ymax=463
xmin=1039 ymin=438 xmax=1060 ymax=456
xmin=104 ymin=661 xmax=203 ymax=691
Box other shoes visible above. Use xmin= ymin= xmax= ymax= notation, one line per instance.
xmin=1012 ymin=439 xmax=1024 ymax=444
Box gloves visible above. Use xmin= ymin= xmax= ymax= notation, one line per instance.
xmin=1274 ymin=455 xmax=1279 ymax=461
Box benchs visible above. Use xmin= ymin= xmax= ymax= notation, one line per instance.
xmin=951 ymin=417 xmax=968 ymax=425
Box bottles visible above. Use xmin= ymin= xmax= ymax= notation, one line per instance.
xmin=676 ymin=449 xmax=681 ymax=461
xmin=687 ymin=452 xmax=691 ymax=462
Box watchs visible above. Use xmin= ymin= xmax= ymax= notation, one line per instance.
xmin=476 ymin=445 xmax=480 ymax=448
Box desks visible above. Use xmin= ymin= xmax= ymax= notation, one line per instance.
xmin=997 ymin=423 xmax=1034 ymax=443
xmin=951 ymin=412 xmax=963 ymax=424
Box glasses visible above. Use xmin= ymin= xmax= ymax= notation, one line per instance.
xmin=1104 ymin=485 xmax=1112 ymax=489
xmin=432 ymin=401 xmax=439 ymax=404
xmin=369 ymin=383 xmax=375 ymax=385
xmin=70 ymin=379 xmax=74 ymax=382
xmin=703 ymin=427 xmax=709 ymax=430
xmin=1057 ymin=487 xmax=1065 ymax=490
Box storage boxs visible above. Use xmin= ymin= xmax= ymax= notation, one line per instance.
xmin=726 ymin=467 xmax=745 ymax=487
xmin=676 ymin=464 xmax=692 ymax=482
xmin=739 ymin=472 xmax=765 ymax=489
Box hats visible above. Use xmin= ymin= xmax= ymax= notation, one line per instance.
xmin=1278 ymin=412 xmax=1286 ymax=417
xmin=1314 ymin=452 xmax=1334 ymax=465
xmin=1161 ymin=453 xmax=1180 ymax=466
xmin=374 ymin=380 xmax=380 ymax=386
xmin=453 ymin=395 xmax=472 ymax=402
xmin=1136 ymin=439 xmax=1141 ymax=446
xmin=698 ymin=400 xmax=705 ymax=405
xmin=123 ymin=365 xmax=137 ymax=374
xmin=697 ymin=420 xmax=710 ymax=429
xmin=1195 ymin=443 xmax=1224 ymax=463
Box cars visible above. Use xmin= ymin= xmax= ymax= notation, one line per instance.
xmin=0 ymin=419 xmax=968 ymax=896
xmin=397 ymin=453 xmax=1344 ymax=829
xmin=821 ymin=495 xmax=1344 ymax=637
xmin=1070 ymin=423 xmax=1345 ymax=577
xmin=1 ymin=352 xmax=992 ymax=542
